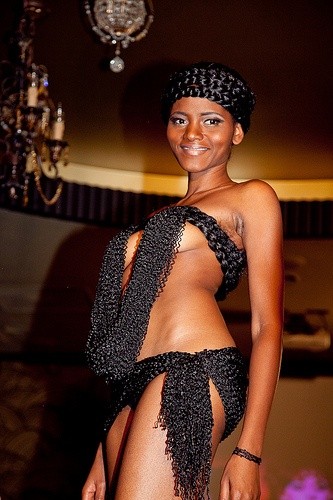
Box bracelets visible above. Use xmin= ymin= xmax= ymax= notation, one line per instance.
xmin=232 ymin=446 xmax=262 ymax=466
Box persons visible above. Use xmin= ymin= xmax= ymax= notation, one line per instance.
xmin=82 ymin=62 xmax=284 ymax=500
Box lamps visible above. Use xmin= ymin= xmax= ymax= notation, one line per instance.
xmin=0 ymin=0 xmax=71 ymax=206
xmin=85 ymin=0 xmax=154 ymax=73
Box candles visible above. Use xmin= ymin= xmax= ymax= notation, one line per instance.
xmin=27 ymin=81 xmax=38 ymax=106
xmin=51 ymin=119 xmax=65 ymax=140
